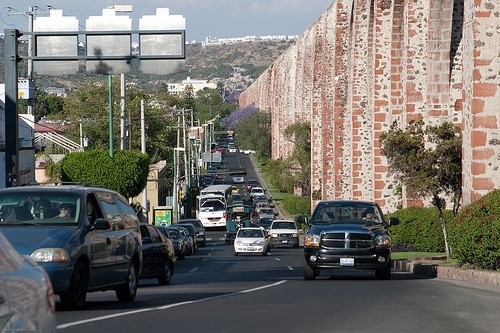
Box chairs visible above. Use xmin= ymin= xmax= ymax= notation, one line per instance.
xmin=323 ymin=212 xmax=335 ymax=220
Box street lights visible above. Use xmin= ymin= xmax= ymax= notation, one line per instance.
xmin=173 ymin=147 xmax=187 ymax=223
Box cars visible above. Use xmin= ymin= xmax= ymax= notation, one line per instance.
xmin=166 ymin=134 xmax=276 ymax=260
xmin=0 ymin=229 xmax=58 ymax=333
xmin=140 ymin=223 xmax=178 ymax=286
xmin=232 ymin=226 xmax=272 ymax=256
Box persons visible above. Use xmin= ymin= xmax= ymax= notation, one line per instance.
xmin=254 ymin=232 xmax=258 ymax=236
xmin=362 ymin=207 xmax=379 ymax=223
xmin=59 ymin=203 xmax=74 ymax=219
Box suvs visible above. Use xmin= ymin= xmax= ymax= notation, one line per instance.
xmin=266 ymin=219 xmax=302 ymax=249
xmin=297 ymin=200 xmax=400 ymax=280
xmin=0 ymin=181 xmax=143 ymax=312
